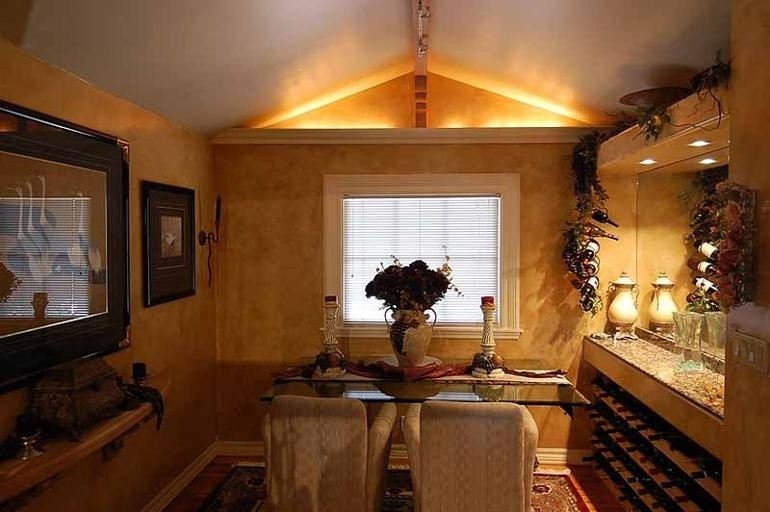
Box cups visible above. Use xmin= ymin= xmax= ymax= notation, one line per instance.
xmin=671 ymin=311 xmax=707 ymax=373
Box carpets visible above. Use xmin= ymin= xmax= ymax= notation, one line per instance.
xmin=194 ymin=459 xmax=600 ymax=512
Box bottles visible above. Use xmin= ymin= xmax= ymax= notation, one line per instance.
xmin=686 ymin=204 xmax=721 ymax=311
xmin=566 ymin=202 xmax=620 ymax=312
xmin=582 ymin=376 xmax=723 ymax=512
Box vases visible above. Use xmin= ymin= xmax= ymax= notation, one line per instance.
xmin=384 ymin=307 xmax=437 ymax=365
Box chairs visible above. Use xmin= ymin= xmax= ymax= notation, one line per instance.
xmin=262 ymin=395 xmax=398 ymax=511
xmin=403 ymin=400 xmax=539 ymax=512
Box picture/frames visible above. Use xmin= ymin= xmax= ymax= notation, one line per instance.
xmin=141 ymin=180 xmax=196 ymax=308
xmin=1 ymin=101 xmax=132 ymax=394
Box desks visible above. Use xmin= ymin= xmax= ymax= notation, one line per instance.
xmin=258 ymin=370 xmax=591 ymax=419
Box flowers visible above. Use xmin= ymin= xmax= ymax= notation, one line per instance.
xmin=365 ymin=244 xmax=464 ymax=311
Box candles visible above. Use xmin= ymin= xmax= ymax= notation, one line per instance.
xmin=324 ymin=296 xmax=338 ymax=306
xmin=481 ymin=297 xmax=495 ymax=307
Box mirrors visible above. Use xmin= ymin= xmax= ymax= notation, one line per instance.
xmin=635 ymin=145 xmax=729 ymax=375
